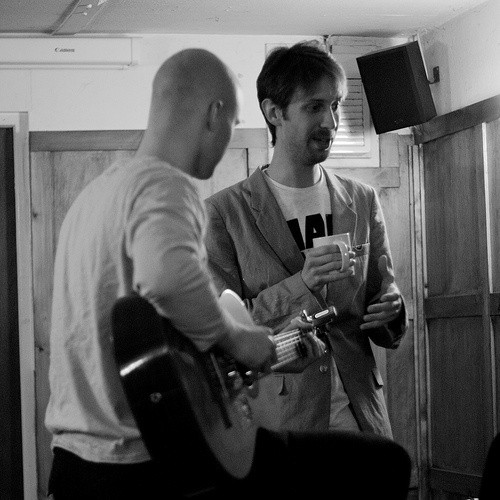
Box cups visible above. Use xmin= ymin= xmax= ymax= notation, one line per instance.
xmin=312 ymin=233 xmax=355 ymax=284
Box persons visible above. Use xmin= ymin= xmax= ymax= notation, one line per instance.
xmin=43 ymin=47 xmax=412 ymax=500
xmin=202 ymin=39 xmax=409 ymax=442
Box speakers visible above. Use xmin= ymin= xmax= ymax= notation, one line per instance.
xmin=355 ymin=40 xmax=437 ymax=135
